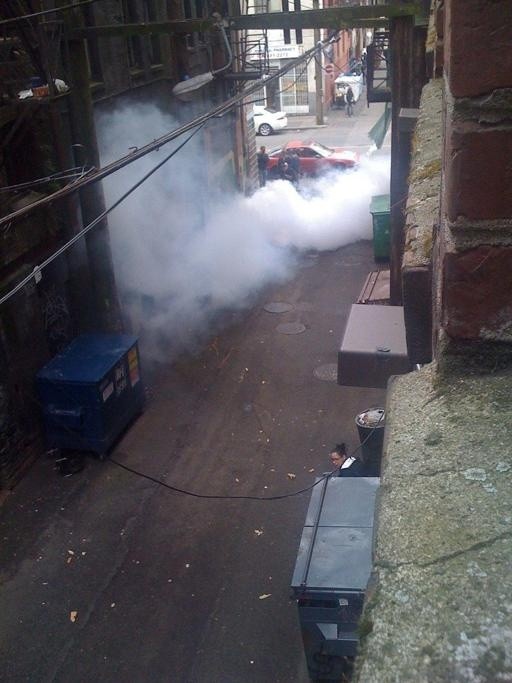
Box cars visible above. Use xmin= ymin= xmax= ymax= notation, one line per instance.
xmin=254 ymin=105 xmax=288 ymax=135
xmin=264 ymin=140 xmax=359 ymax=179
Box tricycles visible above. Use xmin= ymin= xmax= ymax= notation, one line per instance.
xmin=336 ymin=93 xmax=356 ymax=116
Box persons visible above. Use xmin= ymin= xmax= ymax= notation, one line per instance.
xmin=257 ymin=146 xmax=300 ymax=188
xmin=331 ymin=443 xmax=369 ymax=478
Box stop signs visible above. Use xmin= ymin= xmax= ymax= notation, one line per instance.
xmin=323 ymin=64 xmax=334 ymax=75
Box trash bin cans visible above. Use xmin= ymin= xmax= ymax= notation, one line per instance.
xmin=370 ymin=194 xmax=391 ymax=258
